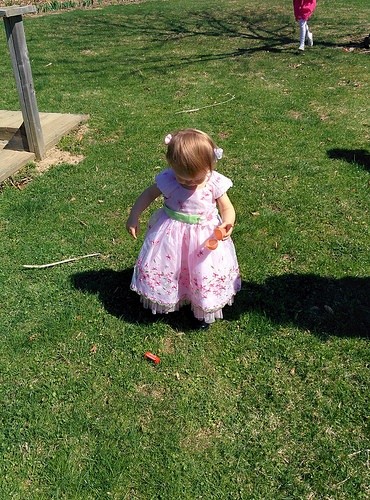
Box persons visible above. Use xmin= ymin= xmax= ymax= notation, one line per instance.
xmin=292 ymin=0 xmax=317 ymax=51
xmin=126 ymin=127 xmax=242 ymax=327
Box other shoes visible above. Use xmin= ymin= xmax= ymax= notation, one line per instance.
xmin=298 ymin=45 xmax=304 ymax=51
xmin=308 ymin=33 xmax=313 ymax=46
